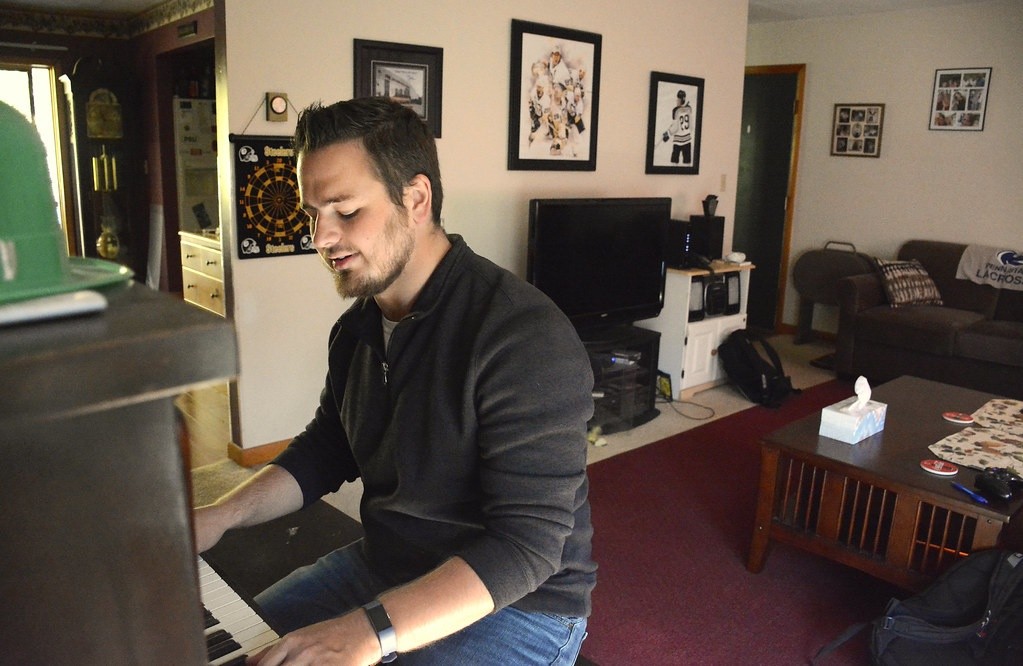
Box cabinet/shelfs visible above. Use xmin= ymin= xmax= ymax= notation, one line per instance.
xmin=579 ymin=323 xmax=661 ymax=435
xmin=178 ymin=231 xmax=226 ymax=318
xmin=633 ymin=261 xmax=755 ymax=400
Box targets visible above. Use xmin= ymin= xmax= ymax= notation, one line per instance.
xmin=230 ymin=135 xmax=318 ymax=260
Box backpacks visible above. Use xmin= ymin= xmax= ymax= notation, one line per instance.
xmin=868 ymin=544 xmax=1023 ymax=666
xmin=718 ymin=328 xmax=803 ymax=405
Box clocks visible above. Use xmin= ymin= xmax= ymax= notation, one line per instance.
xmin=58 ymin=54 xmax=145 ymax=282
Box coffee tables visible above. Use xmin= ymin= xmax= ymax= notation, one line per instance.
xmin=744 ymin=375 xmax=1022 ymax=593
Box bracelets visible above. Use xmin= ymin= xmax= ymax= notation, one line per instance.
xmin=362 ymin=594 xmax=399 ymax=666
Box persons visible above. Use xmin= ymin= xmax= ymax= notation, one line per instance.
xmin=836 ymin=108 xmax=880 ymax=153
xmin=528 ymin=44 xmax=584 ymax=157
xmin=936 ymin=77 xmax=985 ymax=128
xmin=663 ymin=90 xmax=692 ymax=164
xmin=190 ymin=96 xmax=595 ymax=666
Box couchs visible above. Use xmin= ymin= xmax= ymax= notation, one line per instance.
xmin=835 ymin=240 xmax=1023 ymax=401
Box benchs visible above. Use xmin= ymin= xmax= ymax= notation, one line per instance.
xmin=199 ymin=497 xmax=601 ymax=666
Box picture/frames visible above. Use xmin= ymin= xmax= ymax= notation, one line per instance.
xmin=830 ymin=102 xmax=886 ymax=158
xmin=645 ymin=71 xmax=705 ymax=175
xmin=928 ymin=66 xmax=992 ymax=132
xmin=507 ymin=18 xmax=602 ymax=172
xmin=354 ymin=39 xmax=442 ymax=139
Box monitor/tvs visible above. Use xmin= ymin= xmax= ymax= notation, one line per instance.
xmin=526 ymin=198 xmax=672 ymax=342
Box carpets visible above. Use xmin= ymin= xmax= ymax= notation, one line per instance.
xmin=581 ymin=381 xmax=1023 ymax=665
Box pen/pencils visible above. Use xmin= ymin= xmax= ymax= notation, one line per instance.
xmin=948 ymin=481 xmax=988 ymax=504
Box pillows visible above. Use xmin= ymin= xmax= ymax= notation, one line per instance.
xmin=872 ymin=255 xmax=944 ymax=308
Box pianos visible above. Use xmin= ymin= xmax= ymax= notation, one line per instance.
xmin=0 ymin=276 xmax=291 ymax=666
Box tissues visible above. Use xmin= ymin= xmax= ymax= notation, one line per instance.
xmin=818 ymin=375 xmax=887 ymax=445
